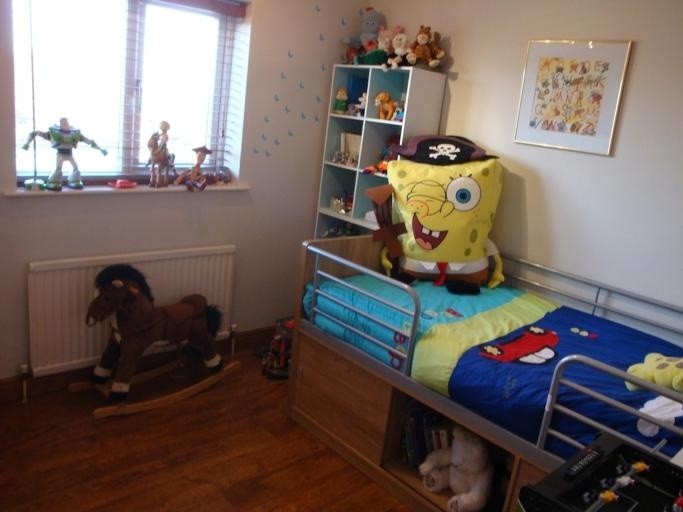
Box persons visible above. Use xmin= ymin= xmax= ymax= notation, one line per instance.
xmin=176 ymin=146 xmax=212 ymax=193
xmin=23 ymin=118 xmax=107 ymax=191
xmin=145 ymin=120 xmax=179 ymax=186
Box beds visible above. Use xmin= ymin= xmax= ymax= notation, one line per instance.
xmin=283 ymin=234 xmax=683 ymax=512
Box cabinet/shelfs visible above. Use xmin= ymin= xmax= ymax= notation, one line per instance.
xmin=312 ymin=63 xmax=447 ymax=239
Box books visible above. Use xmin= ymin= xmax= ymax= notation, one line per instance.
xmin=406 ymin=413 xmax=456 ymax=456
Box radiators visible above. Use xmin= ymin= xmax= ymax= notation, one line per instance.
xmin=28 ymin=242 xmax=236 ymax=379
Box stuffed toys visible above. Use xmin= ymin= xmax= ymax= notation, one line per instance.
xmin=331 ymin=131 xmax=363 ymax=169
xmin=363 ymin=134 xmax=402 ymax=175
xmin=407 ymin=24 xmax=445 ymax=69
xmin=364 ymin=135 xmax=504 ymax=296
xmin=419 ymin=425 xmax=493 ymax=512
xmin=374 ymin=90 xmax=396 ymax=122
xmin=390 ymin=100 xmax=405 ymax=126
xmin=334 ymin=87 xmax=349 ymax=115
xmin=353 ymin=26 xmax=405 ymax=67
xmin=329 ymin=192 xmax=346 ymax=216
xmin=354 ymin=91 xmax=369 ymax=118
xmin=624 ymin=353 xmax=683 ymax=394
xmin=344 ymin=192 xmax=355 ymax=217
xmin=343 ymin=7 xmax=387 ymax=65
xmin=383 ymin=33 xmax=415 ymax=69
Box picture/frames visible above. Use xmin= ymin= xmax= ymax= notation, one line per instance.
xmin=513 ymin=39 xmax=633 ymax=156
